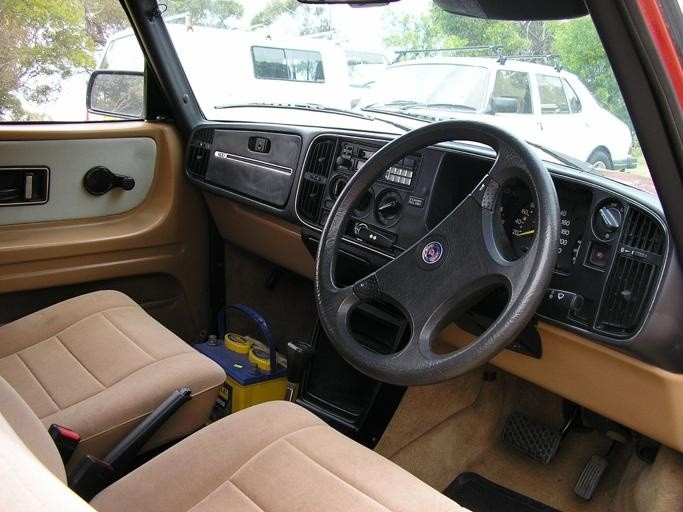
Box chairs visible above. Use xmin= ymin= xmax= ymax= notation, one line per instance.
xmin=0 ymin=376 xmax=475 ymax=512
xmin=0 ymin=288 xmax=226 ymax=481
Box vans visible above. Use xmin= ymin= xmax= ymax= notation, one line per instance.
xmin=86 ymin=14 xmax=393 ymax=129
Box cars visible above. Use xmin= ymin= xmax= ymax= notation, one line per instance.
xmin=353 ymin=45 xmax=639 ymax=180
